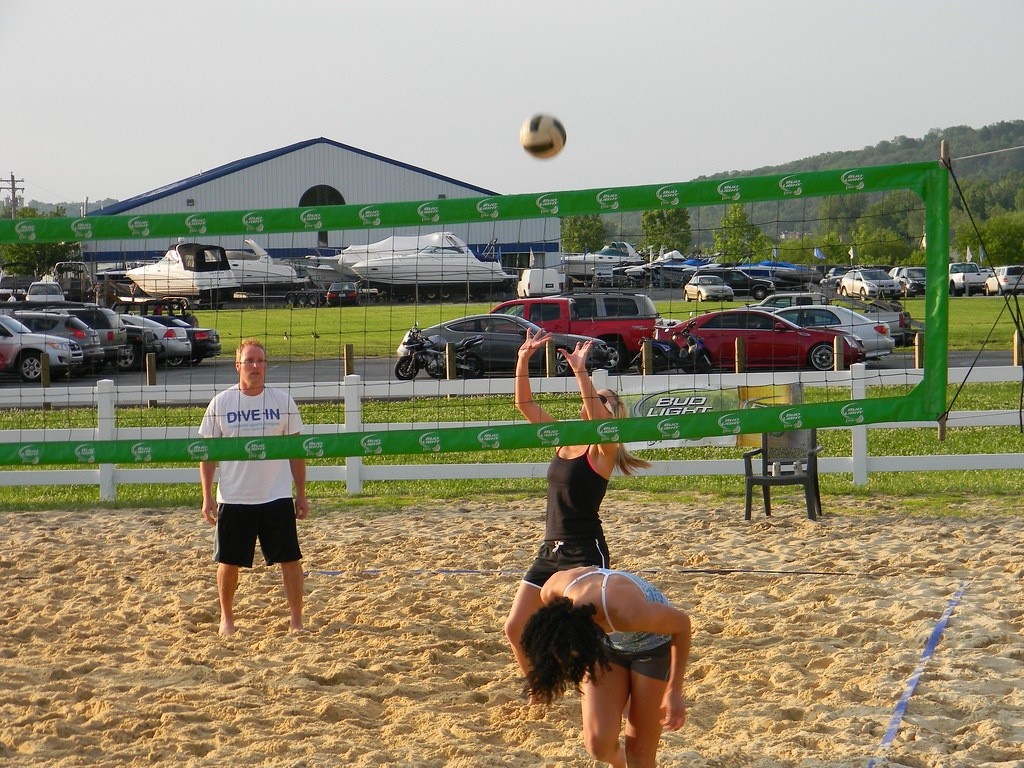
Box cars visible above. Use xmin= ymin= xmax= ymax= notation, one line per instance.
xmin=655 ymin=308 xmax=870 ymax=371
xmin=0 ymin=301 xmax=222 ymax=382
xmin=24 ymin=283 xmax=67 ymax=301
xmin=841 ymin=265 xmax=1024 ymax=299
xmin=326 ymin=282 xmax=361 ymax=306
xmin=684 ymin=275 xmax=734 ymax=301
xmin=752 ymin=305 xmax=895 ymax=360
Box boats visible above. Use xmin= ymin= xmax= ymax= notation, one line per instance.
xmin=53 ymin=262 xmax=93 ymax=300
xmin=560 ymin=242 xmax=823 ymax=282
xmin=0 ymin=263 xmax=40 ymax=300
xmin=298 ymin=232 xmax=519 ymax=288
xmin=224 ymin=239 xmax=298 ymax=283
xmin=95 ymin=242 xmax=241 ymax=300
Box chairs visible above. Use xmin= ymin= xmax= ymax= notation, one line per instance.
xmin=756 ymin=322 xmax=773 ymax=329
xmin=741 ymin=422 xmax=823 ymax=519
xmin=485 ymin=325 xmax=496 ymax=332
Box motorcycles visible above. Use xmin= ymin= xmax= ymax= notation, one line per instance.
xmin=394 ymin=321 xmax=485 ymax=381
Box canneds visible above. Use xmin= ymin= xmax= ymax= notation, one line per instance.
xmin=772 ymin=461 xmax=781 ymax=477
xmin=793 ymin=461 xmax=803 ymax=475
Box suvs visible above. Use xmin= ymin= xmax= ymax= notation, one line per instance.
xmin=509 ymin=290 xmax=661 ymax=322
xmin=693 ymin=269 xmax=776 ymax=299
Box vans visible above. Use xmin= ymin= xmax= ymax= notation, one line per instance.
xmin=518 ymin=269 xmax=561 ymax=297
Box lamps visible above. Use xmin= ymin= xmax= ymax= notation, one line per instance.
xmin=438 ymin=193 xmax=445 ymax=200
xmin=187 ymin=199 xmax=195 ymax=207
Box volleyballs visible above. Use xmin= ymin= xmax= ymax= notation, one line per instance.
xmin=519 ymin=112 xmax=568 ymax=159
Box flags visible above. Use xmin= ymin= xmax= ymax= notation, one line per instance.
xmin=813 ymin=247 xmax=825 ymax=259
xmin=965 ymin=246 xmax=972 ymax=261
xmin=772 ymin=245 xmax=777 ymax=257
xmin=848 ymin=247 xmax=855 ymax=259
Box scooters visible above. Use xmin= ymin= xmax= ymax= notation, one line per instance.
xmin=629 ymin=312 xmax=714 ymax=374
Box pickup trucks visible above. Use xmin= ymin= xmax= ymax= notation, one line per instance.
xmin=819 ymin=267 xmax=858 ymax=295
xmin=949 ymin=261 xmax=992 ymax=297
xmin=464 ymin=298 xmax=664 ymax=373
xmin=759 ymin=292 xmax=914 ymax=344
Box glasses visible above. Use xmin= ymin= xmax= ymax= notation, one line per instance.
xmin=597 ymin=394 xmax=614 ymax=414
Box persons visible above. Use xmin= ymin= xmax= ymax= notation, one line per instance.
xmin=519 ymin=565 xmax=691 ymax=768
xmin=6 ymin=291 xmax=16 ymax=302
xmin=97 ymin=283 xmax=104 ymax=303
xmin=504 ymin=326 xmax=654 ymax=728
xmin=197 ymin=337 xmax=309 ymax=649
